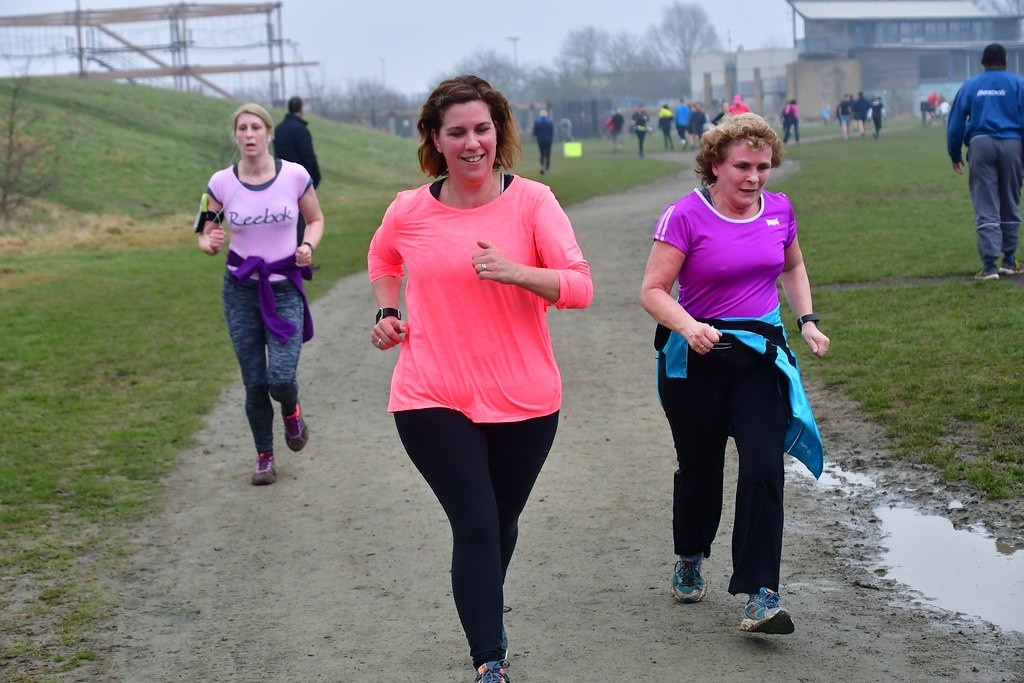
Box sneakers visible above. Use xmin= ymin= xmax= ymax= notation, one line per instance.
xmin=474 ymin=659 xmax=511 ymax=683
xmin=251 ymin=452 xmax=276 ymax=485
xmin=739 ymin=588 xmax=795 ymax=635
xmin=975 ymin=265 xmax=1000 ymax=279
xmin=999 ymin=256 xmax=1024 ymax=274
xmin=501 ymin=605 xmax=512 ymax=664
xmin=282 ymin=397 xmax=308 ymax=452
xmin=670 ymin=554 xmax=707 ymax=603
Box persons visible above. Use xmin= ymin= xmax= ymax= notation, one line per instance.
xmin=607 ymin=106 xmax=625 ymax=151
xmin=198 ymin=103 xmax=325 ymax=487
xmin=631 ymin=104 xmax=650 ymax=156
xmin=853 ymin=90 xmax=872 ymax=136
xmin=838 ymin=93 xmax=852 ymax=139
xmin=532 ymin=109 xmax=554 ymax=174
xmin=642 ymin=113 xmax=831 ymax=635
xmin=711 ymin=99 xmax=729 ymax=125
xmin=784 ymin=101 xmax=801 ymax=144
xmin=947 ymin=43 xmax=1024 ymax=279
xmin=866 ymin=98 xmax=887 ymax=138
xmin=658 ymin=104 xmax=674 ymax=149
xmin=691 ymin=102 xmax=706 ymax=149
xmin=920 ymin=91 xmax=951 ymax=127
xmin=674 ymin=97 xmax=692 ymax=149
xmin=274 ymin=97 xmax=322 ymax=247
xmin=730 ymin=94 xmax=751 ymax=115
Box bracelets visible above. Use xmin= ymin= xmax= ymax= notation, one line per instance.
xmin=303 ymin=242 xmax=313 ymax=254
xmin=797 ymin=313 xmax=820 ymax=332
xmin=376 ymin=308 xmax=402 ymax=325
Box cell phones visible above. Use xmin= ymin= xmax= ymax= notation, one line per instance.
xmin=194 ymin=194 xmax=207 ymax=233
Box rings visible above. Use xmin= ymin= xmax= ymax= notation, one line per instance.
xmin=700 ymin=344 xmax=705 ymax=349
xmin=377 ymin=338 xmax=382 ymax=346
xmin=367 ymin=72 xmax=595 ymax=683
xmin=482 ymin=263 xmax=486 ymax=270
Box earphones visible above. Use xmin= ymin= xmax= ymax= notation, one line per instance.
xmin=268 ymin=135 xmax=271 ymax=142
